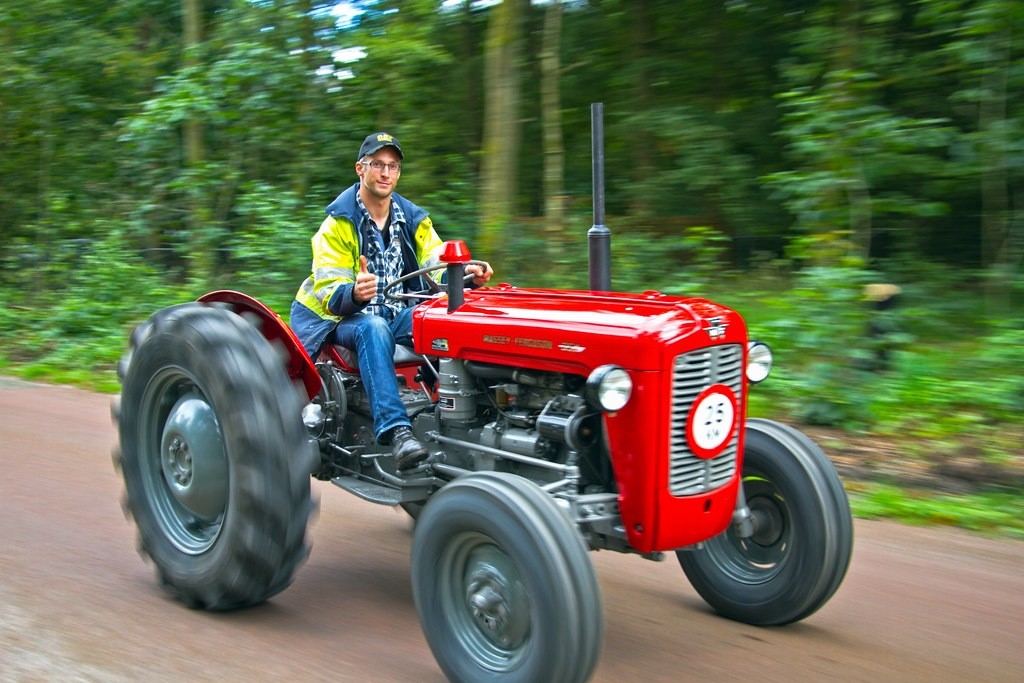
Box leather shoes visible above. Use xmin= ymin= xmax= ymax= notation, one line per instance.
xmin=393 ymin=426 xmax=431 ymax=471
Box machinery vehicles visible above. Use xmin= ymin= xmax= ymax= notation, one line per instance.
xmin=105 ymin=100 xmax=857 ymax=683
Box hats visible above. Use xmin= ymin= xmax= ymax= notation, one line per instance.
xmin=357 ymin=132 xmax=404 ymax=162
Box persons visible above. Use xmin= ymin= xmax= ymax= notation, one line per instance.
xmin=291 ymin=133 xmax=493 ymax=472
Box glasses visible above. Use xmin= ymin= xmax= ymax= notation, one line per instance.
xmin=360 ymin=161 xmax=402 ymax=173
